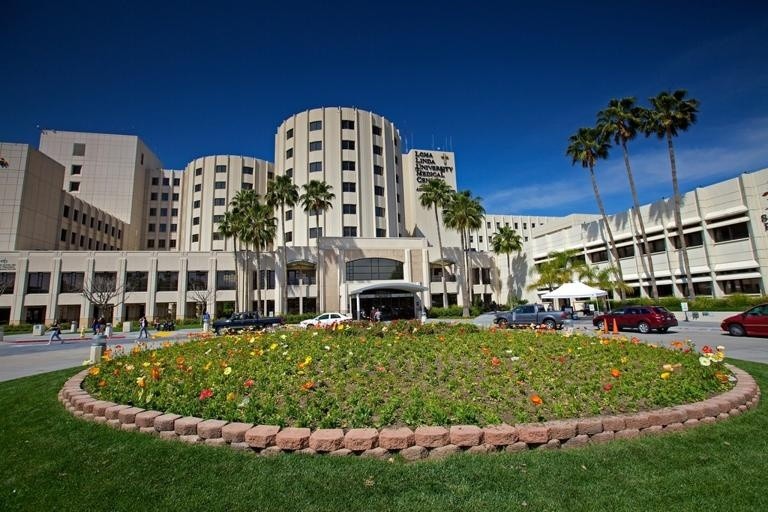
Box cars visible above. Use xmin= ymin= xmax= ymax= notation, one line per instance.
xmin=721 ymin=303 xmax=768 ymax=338
xmin=300 ymin=313 xmax=353 ymax=330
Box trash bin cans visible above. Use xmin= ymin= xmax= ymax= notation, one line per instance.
xmin=203 ymin=319 xmax=209 ymax=332
xmin=105 ymin=323 xmax=112 ymax=338
xmin=90 ymin=335 xmax=106 ymax=362
xmin=33 ymin=325 xmax=44 ymax=335
xmin=70 ymin=321 xmax=77 ymax=332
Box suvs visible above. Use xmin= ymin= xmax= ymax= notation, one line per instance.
xmin=593 ymin=305 xmax=678 ymax=334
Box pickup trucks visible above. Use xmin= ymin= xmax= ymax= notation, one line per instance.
xmin=494 ymin=304 xmax=568 ymax=330
xmin=212 ymin=311 xmax=283 ymax=336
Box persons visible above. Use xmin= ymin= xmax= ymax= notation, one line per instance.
xmin=202 ymin=310 xmax=211 ymax=333
xmin=137 ymin=316 xmax=148 ymax=339
xmin=369 ymin=305 xmax=377 ymax=322
xmin=91 ymin=315 xmax=98 ymax=334
xmin=372 ymin=307 xmax=383 ymax=323
xmin=44 ymin=319 xmax=64 ymax=345
xmin=152 ymin=316 xmax=177 ymax=333
xmin=546 ymin=301 xmax=596 ymax=321
xmin=96 ymin=314 xmax=106 ymax=335
